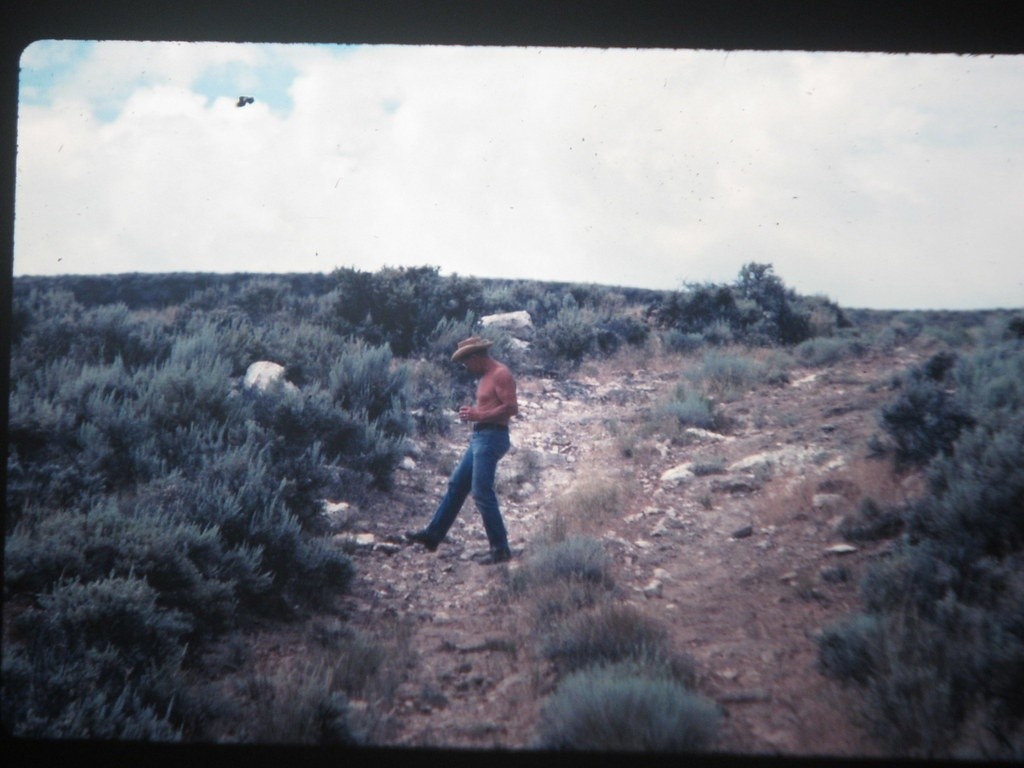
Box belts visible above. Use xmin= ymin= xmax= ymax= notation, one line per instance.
xmin=473 ymin=422 xmax=509 ymax=431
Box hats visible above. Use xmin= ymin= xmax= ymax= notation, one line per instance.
xmin=451 ymin=337 xmax=495 ymax=362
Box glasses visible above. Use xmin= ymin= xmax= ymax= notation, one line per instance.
xmin=462 ymin=361 xmax=469 ymax=369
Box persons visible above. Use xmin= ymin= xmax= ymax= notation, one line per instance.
xmin=402 ymin=336 xmax=520 ymax=565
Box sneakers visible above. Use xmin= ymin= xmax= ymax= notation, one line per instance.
xmin=406 ymin=530 xmax=438 ymax=551
xmin=479 ymin=550 xmax=511 ymax=565
